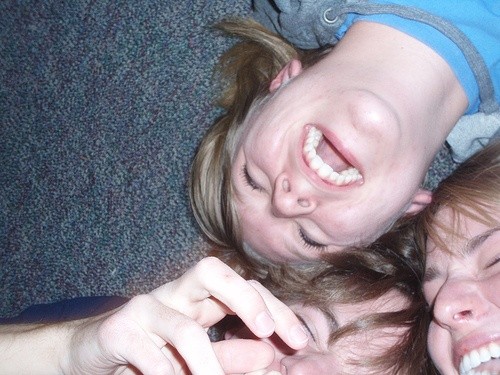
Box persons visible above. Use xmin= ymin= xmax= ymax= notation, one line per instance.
xmin=0 ymin=251 xmax=441 ymax=375
xmin=412 ymin=134 xmax=500 ymax=375
xmin=184 ymin=0 xmax=500 ymax=282
